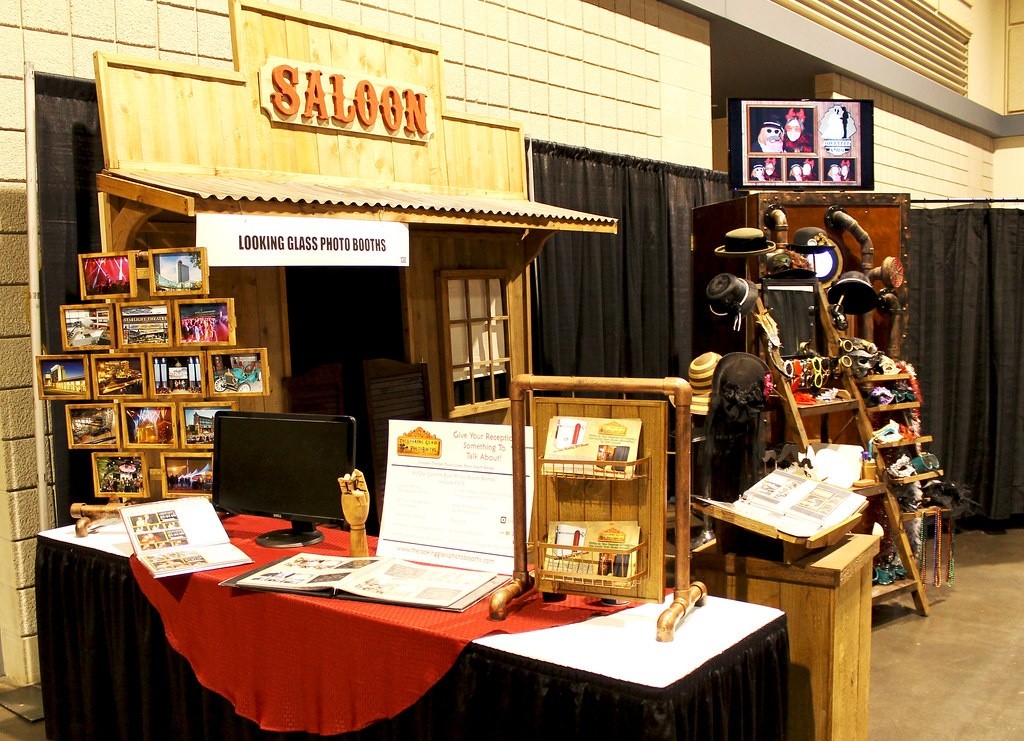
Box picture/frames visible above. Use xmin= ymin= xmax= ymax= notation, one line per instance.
xmin=90 ymin=353 xmax=150 ymax=400
xmin=91 ymin=451 xmax=151 ymax=499
xmin=148 ymin=248 xmax=210 ymax=298
xmin=178 ymin=402 xmax=239 ymax=451
xmin=147 ymin=352 xmax=210 ymax=401
xmin=207 ymin=350 xmax=271 ymax=398
xmin=59 ymin=304 xmax=116 ymax=351
xmin=77 ymin=252 xmax=136 ymax=300
xmin=121 ymin=402 xmax=178 ymax=449
xmin=176 ymin=298 xmax=236 ymax=347
xmin=65 ymin=403 xmax=120 ymax=449
xmin=728 ymin=97 xmax=874 ymax=192
xmin=161 ymin=452 xmax=216 ymax=499
xmin=34 ymin=355 xmax=92 ymax=402
xmin=114 ymin=300 xmax=173 ymax=350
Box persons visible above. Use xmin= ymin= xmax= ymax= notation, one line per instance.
xmin=105 ymin=273 xmax=110 ymax=282
xmin=88 ymin=282 xmax=130 ymax=295
xmin=825 ymin=160 xmax=855 ymax=181
xmin=182 ymin=318 xmax=216 ymax=342
xmin=169 ymin=474 xmax=191 ymax=489
xmin=104 ymin=477 xmax=140 ymax=492
xmin=788 ymin=159 xmax=817 ymax=181
xmin=750 ymin=158 xmax=781 ymax=181
xmin=751 ymin=108 xmax=813 ymax=152
xmin=338 ymin=469 xmax=370 ymax=557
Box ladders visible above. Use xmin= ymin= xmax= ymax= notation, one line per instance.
xmin=747 ymin=278 xmax=930 ymax=618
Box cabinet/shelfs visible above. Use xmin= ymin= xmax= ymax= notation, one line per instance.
xmin=768 ymin=356 xmax=951 ymax=617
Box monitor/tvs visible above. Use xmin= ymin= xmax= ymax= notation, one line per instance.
xmin=212 ymin=411 xmax=356 ymax=548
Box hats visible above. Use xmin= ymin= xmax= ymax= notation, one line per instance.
xmin=826 ymin=271 xmax=878 ymax=315
xmin=668 ymin=351 xmax=723 ymax=415
xmin=706 ymin=273 xmax=758 ymax=332
xmin=714 ymin=227 xmax=777 ymax=259
xmin=762 ymin=116 xmax=784 ymax=131
xmin=711 ymin=352 xmax=773 ymax=416
xmin=752 ymin=160 xmax=763 ymax=169
xmin=763 ymin=254 xmax=817 ymax=279
xmin=786 ymin=227 xmax=836 ymax=254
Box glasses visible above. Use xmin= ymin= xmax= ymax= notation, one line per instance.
xmin=785 ymin=125 xmax=802 ymax=133
xmin=765 ymin=167 xmax=775 ymax=171
xmin=766 ymin=128 xmax=780 ymax=135
xmin=910 ymin=454 xmax=940 ymax=473
xmin=784 ymin=338 xmax=853 ymax=390
xmin=756 ymin=168 xmax=763 ymax=171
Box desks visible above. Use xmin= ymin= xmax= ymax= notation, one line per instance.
xmin=688 ymin=534 xmax=881 ymax=741
xmin=37 ymin=512 xmax=789 ymax=741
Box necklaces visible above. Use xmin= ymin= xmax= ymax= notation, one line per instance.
xmin=919 ymin=511 xmax=927 ymax=592
xmin=946 ymin=518 xmax=956 ymax=587
xmin=932 ymin=508 xmax=942 ymax=587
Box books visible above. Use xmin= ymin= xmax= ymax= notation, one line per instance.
xmin=118 ymin=497 xmax=252 ymax=579
xmin=220 ymin=552 xmax=513 ymax=612
xmin=700 ymin=469 xmax=867 ymax=537
